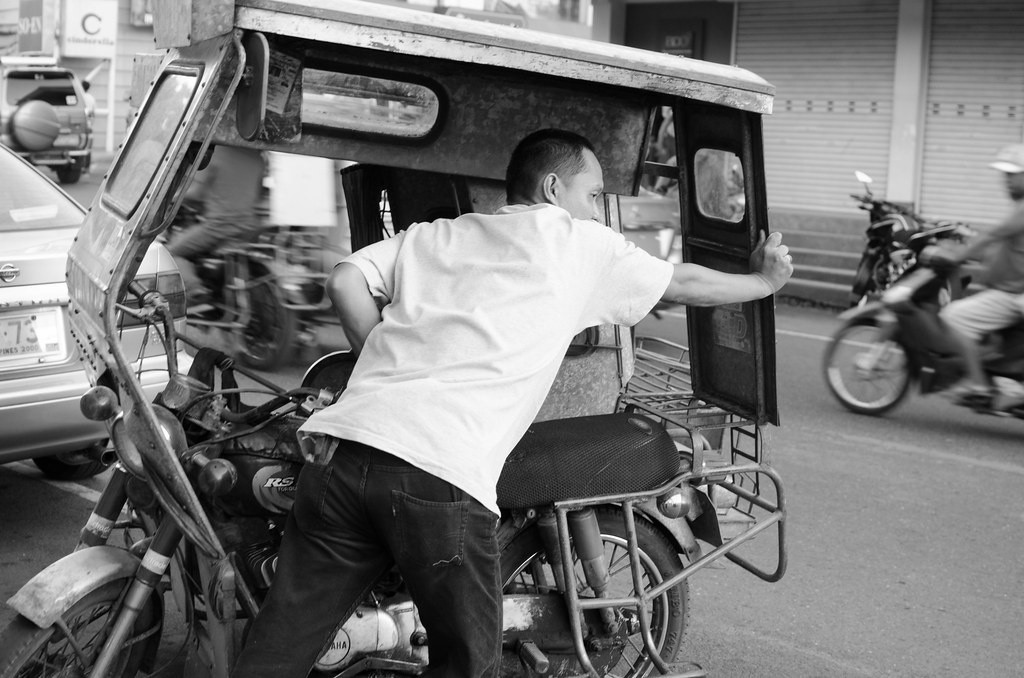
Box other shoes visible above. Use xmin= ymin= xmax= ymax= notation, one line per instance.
xmin=185 ymin=293 xmax=215 ymax=314
xmin=939 ymin=380 xmax=996 ymax=401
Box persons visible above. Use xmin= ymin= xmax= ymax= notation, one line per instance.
xmin=165 ymin=142 xmax=264 ymax=311
xmin=79 ymin=82 xmax=96 ymax=173
xmin=933 ymin=145 xmax=1024 ymax=404
xmin=228 ymin=108 xmax=795 ymax=678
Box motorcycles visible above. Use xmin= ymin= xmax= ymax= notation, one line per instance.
xmin=0 ymin=1 xmax=785 ymax=678
xmin=167 ymin=215 xmax=339 ymax=372
xmin=821 ymin=222 xmax=1024 ymax=425
xmin=848 ymin=169 xmax=968 ymax=309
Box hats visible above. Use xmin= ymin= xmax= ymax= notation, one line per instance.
xmin=987 ymin=144 xmax=1024 ymax=173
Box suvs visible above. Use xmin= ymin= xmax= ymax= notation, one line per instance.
xmin=0 ymin=48 xmax=92 ymax=193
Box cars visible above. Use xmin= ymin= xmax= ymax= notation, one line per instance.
xmin=0 ymin=141 xmax=187 ymax=482
